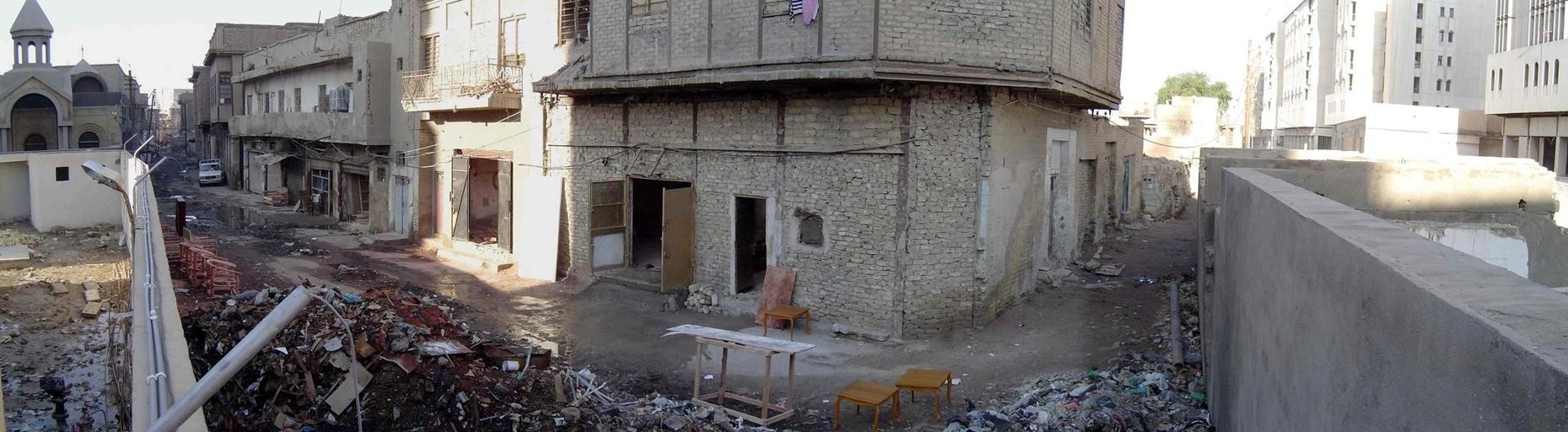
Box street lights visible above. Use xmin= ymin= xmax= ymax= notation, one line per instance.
xmin=178 ymin=103 xmax=187 ymax=156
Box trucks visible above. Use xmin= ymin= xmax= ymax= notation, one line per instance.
xmin=198 ymin=158 xmax=222 ymax=186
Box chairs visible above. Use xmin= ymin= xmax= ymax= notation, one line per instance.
xmin=295 ymin=195 xmax=324 ymax=216
xmin=179 ymin=226 xmax=242 ymax=298
xmin=264 ymin=187 xmax=290 ymax=206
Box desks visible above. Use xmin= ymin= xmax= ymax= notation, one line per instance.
xmin=690 ymin=335 xmax=796 ymax=427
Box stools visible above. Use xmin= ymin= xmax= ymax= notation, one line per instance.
xmin=762 ymin=305 xmax=810 ymax=341
xmin=833 ymin=378 xmax=900 ymax=432
xmin=894 ymin=370 xmax=952 ymax=422
